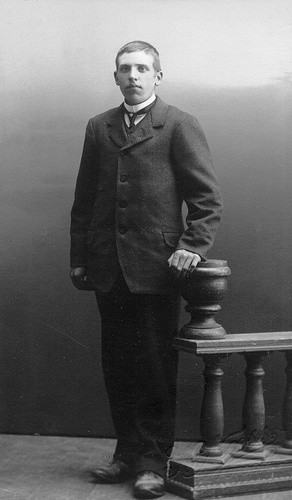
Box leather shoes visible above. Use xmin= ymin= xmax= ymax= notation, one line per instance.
xmin=89 ymin=462 xmax=130 ymax=483
xmin=134 ymin=472 xmax=165 ymax=498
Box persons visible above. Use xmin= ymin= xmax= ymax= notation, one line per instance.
xmin=70 ymin=39 xmax=224 ymax=497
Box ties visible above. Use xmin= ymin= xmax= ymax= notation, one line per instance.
xmin=121 ymin=99 xmax=157 ymax=128
